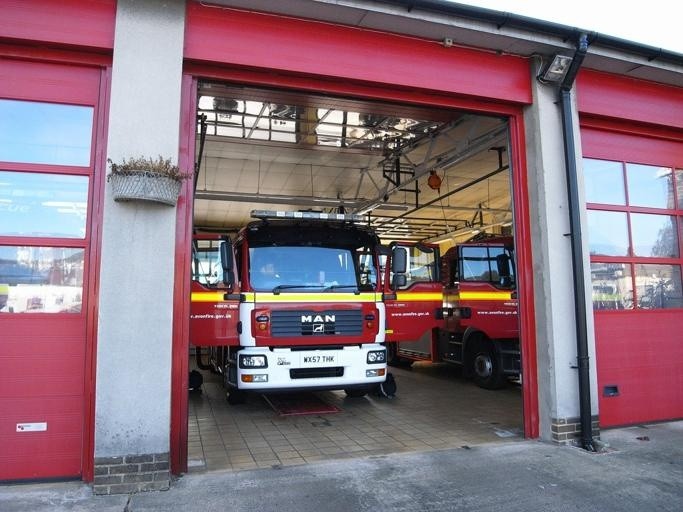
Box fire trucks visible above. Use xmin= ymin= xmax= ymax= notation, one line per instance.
xmin=386 ymin=227 xmax=519 ymax=392
xmin=188 ymin=209 xmax=444 ymax=406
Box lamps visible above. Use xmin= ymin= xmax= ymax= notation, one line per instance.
xmin=542 ymin=51 xmax=574 ymax=84
